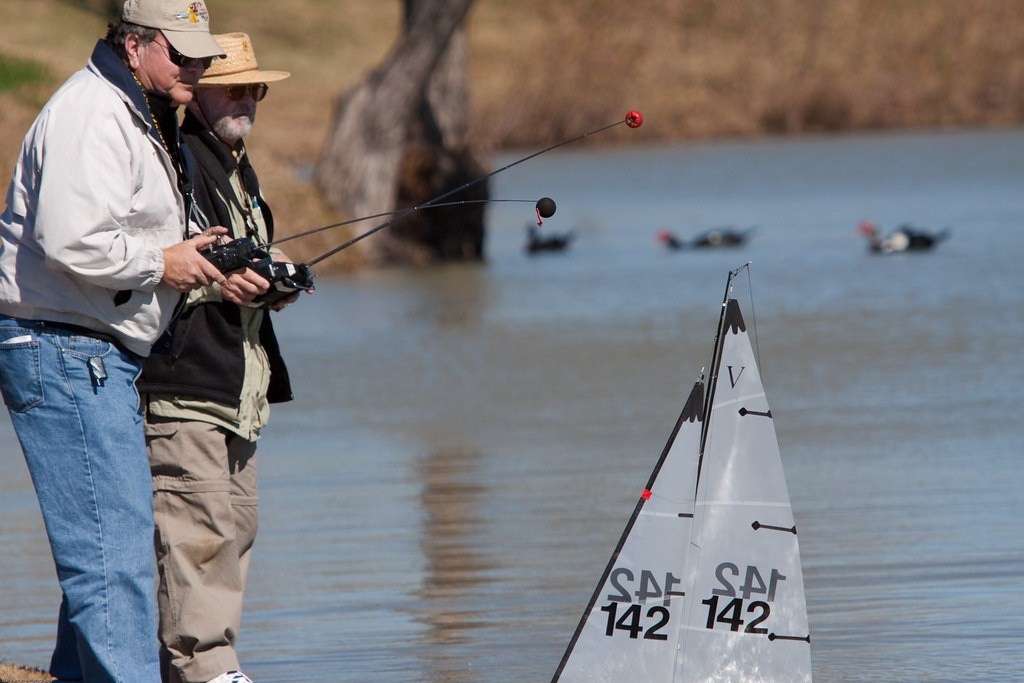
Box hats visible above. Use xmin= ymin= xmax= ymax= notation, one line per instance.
xmin=122 ymin=0 xmax=227 ymax=60
xmin=193 ymin=32 xmax=291 ymax=87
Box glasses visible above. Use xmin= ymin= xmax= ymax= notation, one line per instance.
xmin=226 ymin=82 xmax=269 ymax=101
xmin=137 ymin=34 xmax=212 ymax=69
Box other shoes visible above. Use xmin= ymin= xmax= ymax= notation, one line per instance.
xmin=206 ymin=670 xmax=253 ymax=683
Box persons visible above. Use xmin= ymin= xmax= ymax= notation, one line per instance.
xmin=134 ymin=32 xmax=315 ymax=683
xmin=0 ymin=0 xmax=247 ymax=683
